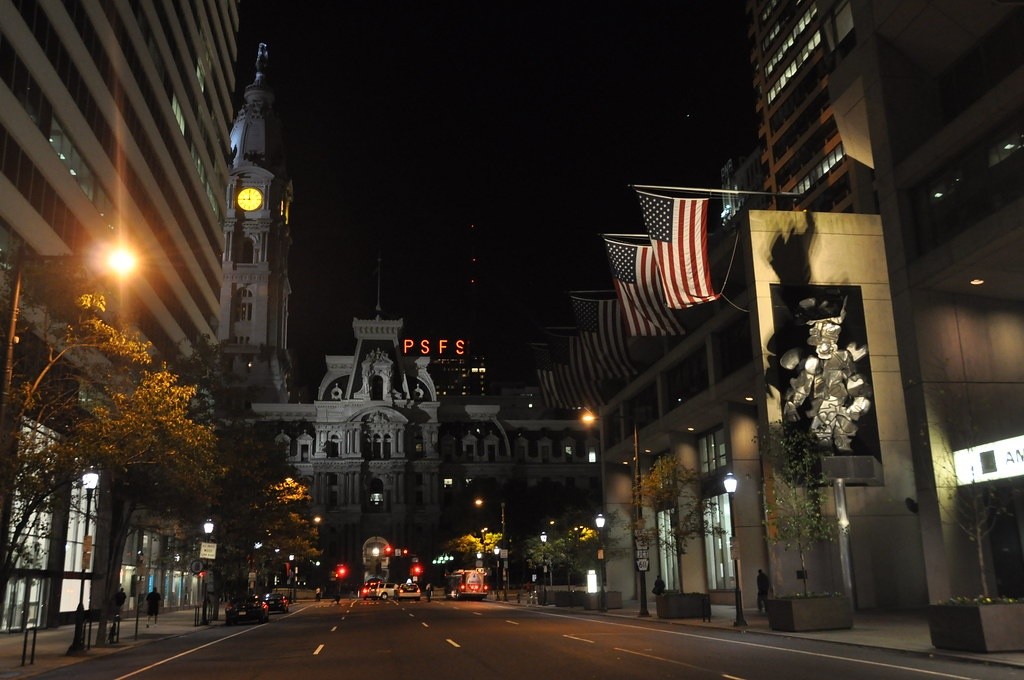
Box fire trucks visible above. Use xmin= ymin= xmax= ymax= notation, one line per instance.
xmin=440 ymin=568 xmax=489 ymax=600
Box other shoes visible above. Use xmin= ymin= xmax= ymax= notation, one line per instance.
xmin=154 ymin=624 xmax=158 ymax=627
xmin=146 ymin=623 xmax=149 ymax=628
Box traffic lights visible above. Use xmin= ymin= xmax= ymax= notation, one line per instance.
xmin=385 ymin=547 xmax=390 ymax=557
xmin=415 ymin=568 xmax=419 ymax=572
xmin=404 ymin=549 xmax=407 ymax=554
xmin=340 ymin=569 xmax=344 ymax=574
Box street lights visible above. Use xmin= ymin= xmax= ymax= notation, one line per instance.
xmin=583 ymin=415 xmax=650 ymax=616
xmin=596 ymin=513 xmax=607 ymax=613
xmin=66 ymin=466 xmax=99 ymax=656
xmin=540 ymin=531 xmax=548 ymax=606
xmin=200 ymin=519 xmax=214 ymax=625
xmin=476 ymin=500 xmax=508 ymax=602
xmin=1 ymin=249 xmax=132 ymax=444
xmin=723 ymin=472 xmax=748 ymax=626
xmin=494 ymin=546 xmax=500 ymax=601
xmin=288 ymin=555 xmax=294 ymax=604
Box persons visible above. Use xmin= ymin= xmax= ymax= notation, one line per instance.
xmin=314 ymin=586 xmax=320 ymax=602
xmin=146 ymin=587 xmax=161 ymax=628
xmin=426 ymin=583 xmax=432 ymax=603
xmin=757 ymin=569 xmax=770 ymax=616
xmin=652 ymin=576 xmax=666 ymax=596
xmin=113 ymin=587 xmax=126 ymax=622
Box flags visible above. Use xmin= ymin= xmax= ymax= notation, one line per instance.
xmin=533 ymin=295 xmax=639 ymax=411
xmin=635 ymin=191 xmax=721 ymax=310
xmin=605 ymin=239 xmax=686 ymax=338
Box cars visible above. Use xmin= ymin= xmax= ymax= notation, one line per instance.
xmin=358 ymin=583 xmax=399 ymax=600
xmin=262 ymin=593 xmax=290 ymax=613
xmin=224 ymin=595 xmax=269 ymax=624
xmin=398 ymin=583 xmax=421 ymax=600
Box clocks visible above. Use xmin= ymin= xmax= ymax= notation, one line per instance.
xmin=237 ymin=187 xmax=263 ymax=211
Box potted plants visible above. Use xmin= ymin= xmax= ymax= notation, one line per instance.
xmin=523 ymin=508 xmax=623 ymax=609
xmin=635 ymin=452 xmax=716 ymax=618
xmin=753 ymin=415 xmax=856 ymax=633
xmin=903 ymin=385 xmax=1024 ymax=657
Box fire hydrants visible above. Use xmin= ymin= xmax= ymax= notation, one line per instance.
xmin=517 ymin=593 xmax=521 ymax=603
